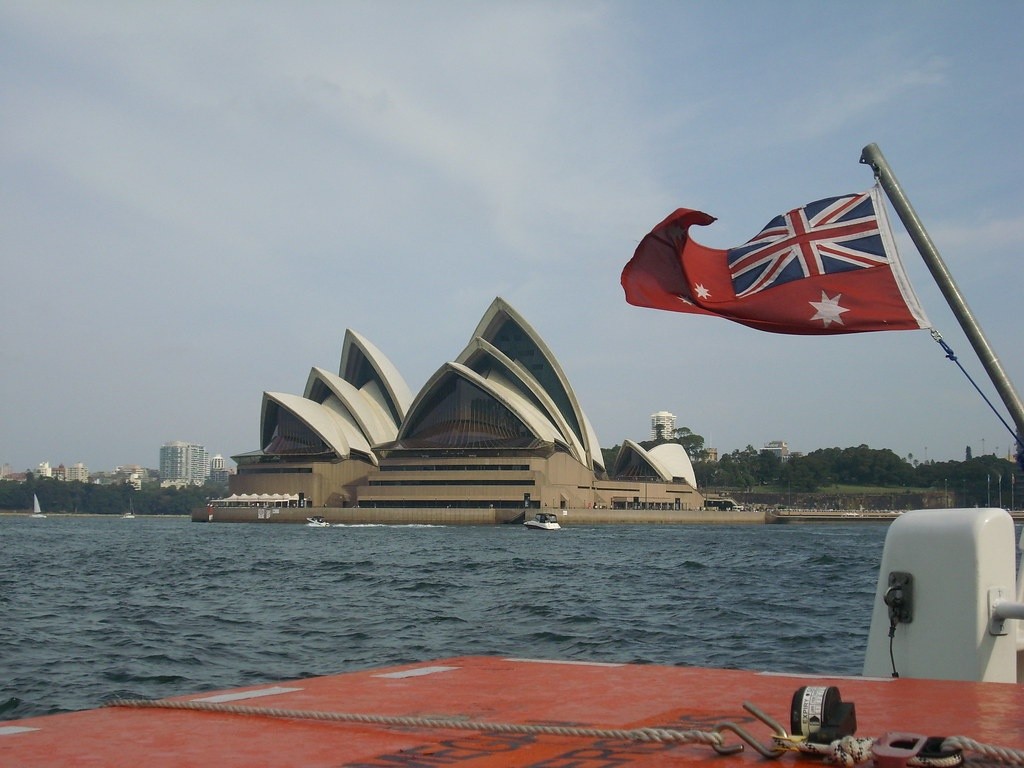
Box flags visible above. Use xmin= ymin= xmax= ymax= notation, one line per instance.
xmin=617 ymin=177 xmax=933 ymax=337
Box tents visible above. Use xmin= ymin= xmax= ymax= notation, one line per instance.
xmin=210 ymin=492 xmax=306 ymax=508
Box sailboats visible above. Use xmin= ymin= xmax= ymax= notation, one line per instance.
xmin=118 ymin=500 xmax=137 ymax=518
xmin=31 ymin=492 xmax=49 ymax=517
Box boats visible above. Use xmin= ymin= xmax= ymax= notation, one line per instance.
xmin=524 ymin=513 xmax=563 ymax=530
xmin=304 ymin=516 xmax=330 ymax=527
